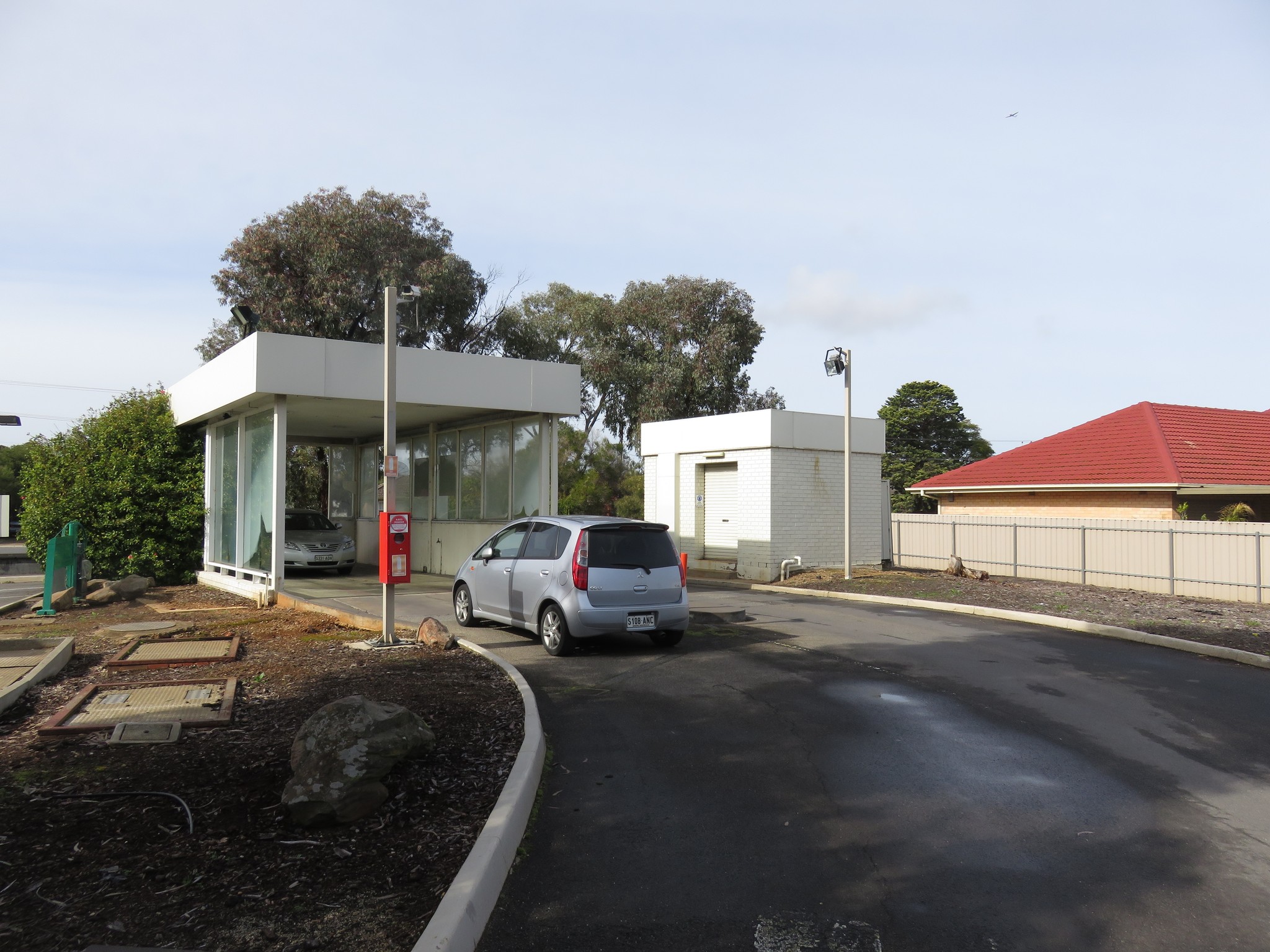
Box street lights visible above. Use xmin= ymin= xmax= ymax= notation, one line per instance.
xmin=824 ymin=347 xmax=852 ymax=579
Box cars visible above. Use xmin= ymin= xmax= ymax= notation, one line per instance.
xmin=285 ymin=508 xmax=356 ymax=574
xmin=452 ymin=515 xmax=689 ymax=656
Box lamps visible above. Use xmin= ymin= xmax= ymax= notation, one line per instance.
xmin=230 ymin=304 xmax=260 ymax=341
